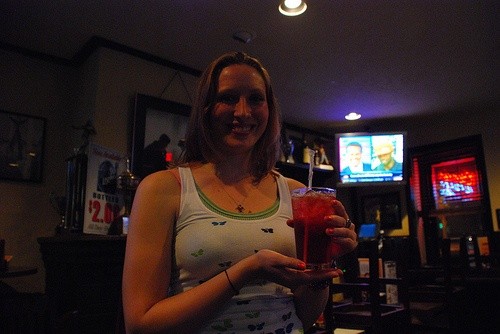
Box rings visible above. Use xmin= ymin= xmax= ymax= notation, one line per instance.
xmin=345 ymin=218 xmax=351 ymax=228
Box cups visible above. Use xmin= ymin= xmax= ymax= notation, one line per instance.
xmin=290 ymin=187 xmax=337 ymax=273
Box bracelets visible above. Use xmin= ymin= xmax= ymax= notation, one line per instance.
xmin=224 ymin=270 xmax=240 ymax=296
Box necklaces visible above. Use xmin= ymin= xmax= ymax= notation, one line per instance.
xmin=220 ymin=183 xmax=252 ymax=212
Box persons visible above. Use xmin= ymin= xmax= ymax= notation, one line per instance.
xmin=341 ymin=142 xmax=370 ymax=173
xmin=122 ymin=51 xmax=357 ymax=334
xmin=71 ymin=120 xmax=97 ymax=153
xmin=319 ymin=143 xmax=329 ymax=165
xmin=373 ymin=140 xmax=402 ymax=171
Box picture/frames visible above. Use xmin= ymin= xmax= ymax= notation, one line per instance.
xmin=0 ymin=108 xmax=48 ymax=184
xmin=130 ymin=93 xmax=193 ymax=178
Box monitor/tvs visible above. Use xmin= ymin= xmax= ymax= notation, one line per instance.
xmin=358 ymin=224 xmax=378 ymax=239
xmin=336 ymin=132 xmax=408 ymax=187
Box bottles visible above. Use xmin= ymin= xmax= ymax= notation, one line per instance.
xmin=278 ymin=131 xmax=312 ymax=167
xmin=110 ymin=158 xmax=136 ymax=235
xmin=374 ymin=209 xmax=383 ymax=239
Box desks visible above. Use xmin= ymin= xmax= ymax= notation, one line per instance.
xmin=35 ymin=233 xmax=128 ymax=334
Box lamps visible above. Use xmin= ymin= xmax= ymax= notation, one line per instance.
xmin=277 ymin=0 xmax=308 ymax=17
